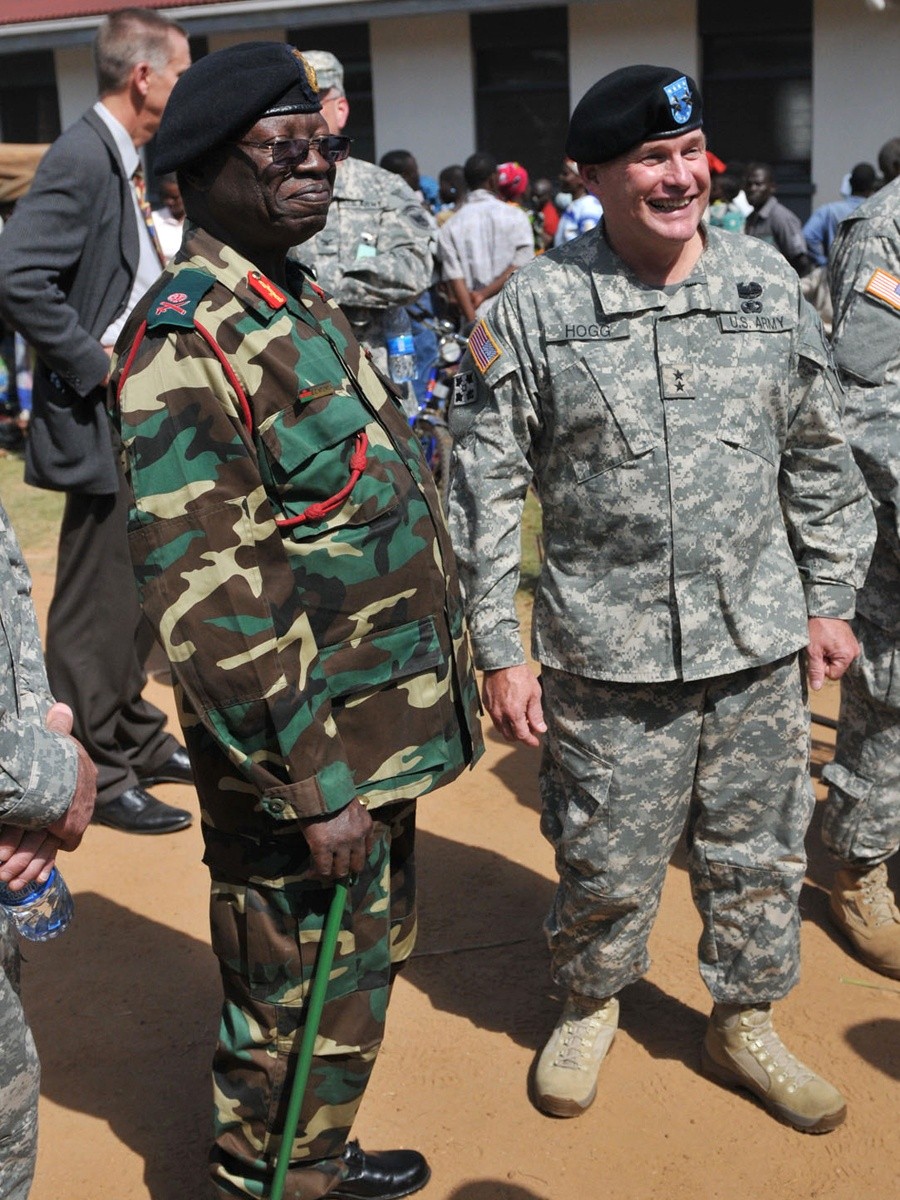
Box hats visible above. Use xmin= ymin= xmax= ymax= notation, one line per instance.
xmin=563 ymin=65 xmax=705 ymax=165
xmin=301 ymin=49 xmax=346 ymax=94
xmin=149 ymin=40 xmax=325 ymax=176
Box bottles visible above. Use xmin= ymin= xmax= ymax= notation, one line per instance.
xmin=0 ymin=862 xmax=75 ymax=942
xmin=384 ymin=307 xmax=418 ymax=385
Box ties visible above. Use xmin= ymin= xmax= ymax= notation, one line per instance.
xmin=134 ymin=164 xmax=167 ymax=269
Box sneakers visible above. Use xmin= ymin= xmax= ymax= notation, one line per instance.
xmin=696 ymin=998 xmax=851 ymax=1137
xmin=827 ymin=860 xmax=900 ymax=979
xmin=534 ymin=992 xmax=620 ymax=1119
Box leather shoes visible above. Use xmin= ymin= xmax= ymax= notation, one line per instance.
xmin=137 ymin=744 xmax=196 ymax=790
xmin=325 ymin=1136 xmax=432 ymax=1200
xmin=90 ymin=785 xmax=193 ymax=834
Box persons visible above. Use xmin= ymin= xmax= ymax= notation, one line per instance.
xmin=291 ymin=47 xmax=900 ymax=434
xmin=0 ymin=7 xmax=197 ymax=835
xmin=823 ymin=173 xmax=900 ymax=981
xmin=447 ymin=66 xmax=878 ymax=1133
xmin=104 ymin=39 xmax=487 ymax=1200
xmin=0 ymin=502 xmax=100 ymax=1200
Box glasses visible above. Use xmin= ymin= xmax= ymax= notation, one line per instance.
xmin=238 ymin=132 xmax=353 ymax=169
